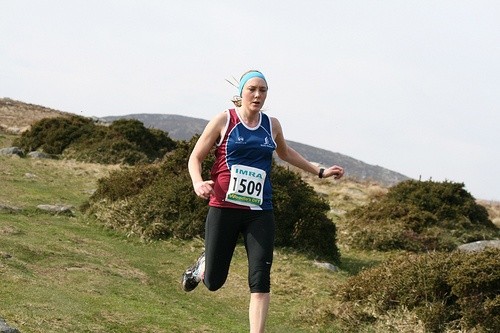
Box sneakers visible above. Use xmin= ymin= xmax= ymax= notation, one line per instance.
xmin=182 ymin=251 xmax=206 ymax=292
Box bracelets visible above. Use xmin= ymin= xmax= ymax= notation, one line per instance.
xmin=318 ymin=168 xmax=325 ymax=178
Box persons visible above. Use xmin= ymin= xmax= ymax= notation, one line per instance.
xmin=180 ymin=69 xmax=346 ymax=333
